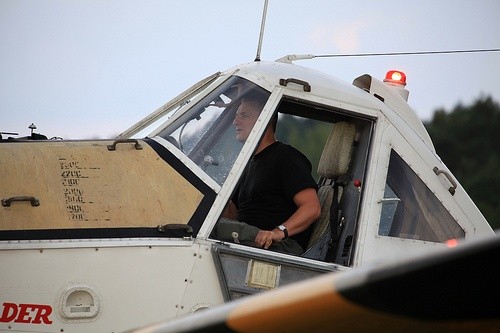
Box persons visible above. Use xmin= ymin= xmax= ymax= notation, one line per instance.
xmin=214 ymin=90 xmax=322 ymax=256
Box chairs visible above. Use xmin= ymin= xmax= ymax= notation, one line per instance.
xmin=289 ymin=119 xmax=356 ymax=261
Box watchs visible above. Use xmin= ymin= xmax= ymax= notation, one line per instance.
xmin=277 ymin=224 xmax=289 ymax=240
